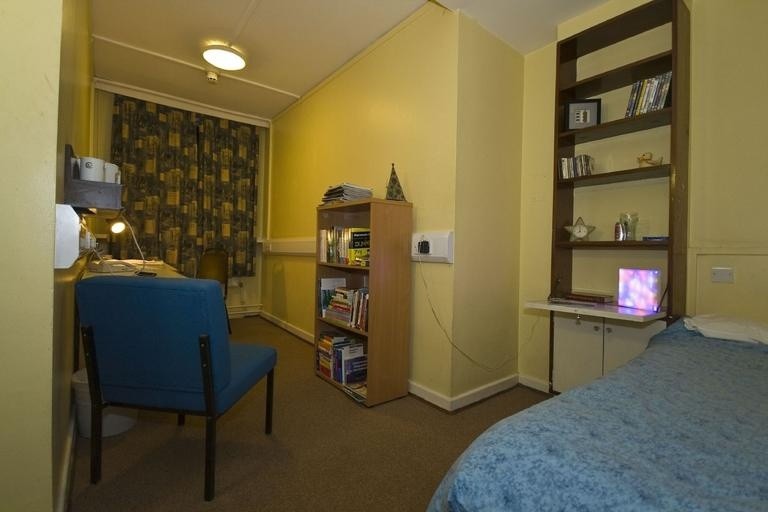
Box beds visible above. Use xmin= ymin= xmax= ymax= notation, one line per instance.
xmin=445 ymin=243 xmax=768 ymax=512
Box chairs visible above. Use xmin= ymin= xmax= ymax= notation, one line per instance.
xmin=195 ymin=247 xmax=232 ymax=334
xmin=74 ymin=276 xmax=277 ymax=502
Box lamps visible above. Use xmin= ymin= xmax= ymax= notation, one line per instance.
xmin=105 ymin=210 xmax=145 ymax=276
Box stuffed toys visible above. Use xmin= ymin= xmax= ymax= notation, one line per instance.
xmin=632 ymin=150 xmax=664 ymax=169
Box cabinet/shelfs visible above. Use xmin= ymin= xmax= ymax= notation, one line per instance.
xmin=524 ymin=1 xmax=689 ymax=395
xmin=315 ymin=199 xmax=414 ymax=407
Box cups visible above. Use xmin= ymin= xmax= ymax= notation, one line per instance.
xmin=620 ymin=211 xmax=639 ymax=241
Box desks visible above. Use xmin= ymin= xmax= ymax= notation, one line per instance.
xmin=73 ymin=256 xmax=188 ymax=373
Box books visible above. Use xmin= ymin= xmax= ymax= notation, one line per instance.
xmin=558 ymin=153 xmax=592 ymax=179
xmin=624 ymin=72 xmax=672 ymax=119
xmin=313 ymin=221 xmax=371 ymax=405
xmin=320 ymin=183 xmax=373 ymax=205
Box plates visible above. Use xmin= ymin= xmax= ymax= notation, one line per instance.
xmin=643 ymin=235 xmax=669 ymax=240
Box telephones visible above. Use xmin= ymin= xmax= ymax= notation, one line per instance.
xmin=87 ymin=259 xmax=137 ymax=273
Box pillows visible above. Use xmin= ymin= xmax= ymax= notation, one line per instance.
xmin=683 ymin=314 xmax=768 ymax=347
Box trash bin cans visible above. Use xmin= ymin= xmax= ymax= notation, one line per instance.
xmin=72 ymin=367 xmax=137 ymax=438
